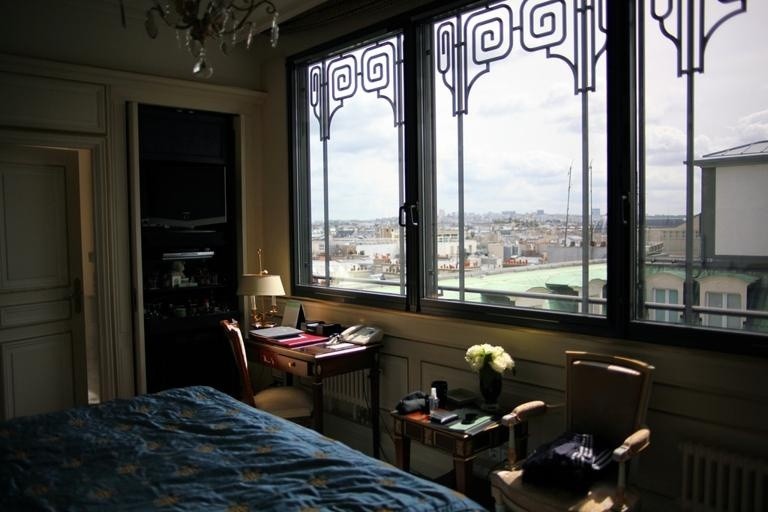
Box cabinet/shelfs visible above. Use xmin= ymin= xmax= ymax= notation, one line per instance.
xmin=127 ymin=101 xmax=242 ymax=402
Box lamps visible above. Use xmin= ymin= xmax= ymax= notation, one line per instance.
xmin=236 ymin=248 xmax=285 ymax=329
xmin=142 ymin=0 xmax=280 ymax=82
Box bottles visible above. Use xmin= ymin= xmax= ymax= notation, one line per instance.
xmin=428 ymin=387 xmax=441 ymax=417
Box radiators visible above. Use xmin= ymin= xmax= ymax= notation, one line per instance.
xmin=676 ymin=440 xmax=768 ymax=512
xmin=322 ymin=368 xmax=371 ymax=421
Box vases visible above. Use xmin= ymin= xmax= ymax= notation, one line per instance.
xmin=479 ymin=362 xmax=502 ymax=411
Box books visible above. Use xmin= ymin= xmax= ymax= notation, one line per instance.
xmin=249 ymin=326 xmax=304 ymax=340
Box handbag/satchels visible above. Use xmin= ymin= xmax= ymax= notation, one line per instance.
xmin=522 ymin=432 xmax=613 ymax=499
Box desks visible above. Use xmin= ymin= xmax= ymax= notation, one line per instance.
xmin=244 ymin=333 xmax=382 ymax=460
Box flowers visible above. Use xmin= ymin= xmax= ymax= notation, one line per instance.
xmin=464 ymin=341 xmax=517 ymax=377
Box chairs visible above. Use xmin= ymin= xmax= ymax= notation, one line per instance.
xmin=220 ymin=319 xmax=315 ymax=430
xmin=488 ymin=350 xmax=655 ymax=512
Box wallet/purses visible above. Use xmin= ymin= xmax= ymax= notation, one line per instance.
xmin=430 ymin=412 xmax=458 ymax=424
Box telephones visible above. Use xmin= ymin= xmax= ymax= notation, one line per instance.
xmin=340 ymin=324 xmax=384 ymax=345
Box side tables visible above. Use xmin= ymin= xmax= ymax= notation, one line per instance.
xmin=389 ymin=400 xmax=529 ymax=498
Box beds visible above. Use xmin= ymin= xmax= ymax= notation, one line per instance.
xmin=0 ymin=385 xmax=488 ymax=512
